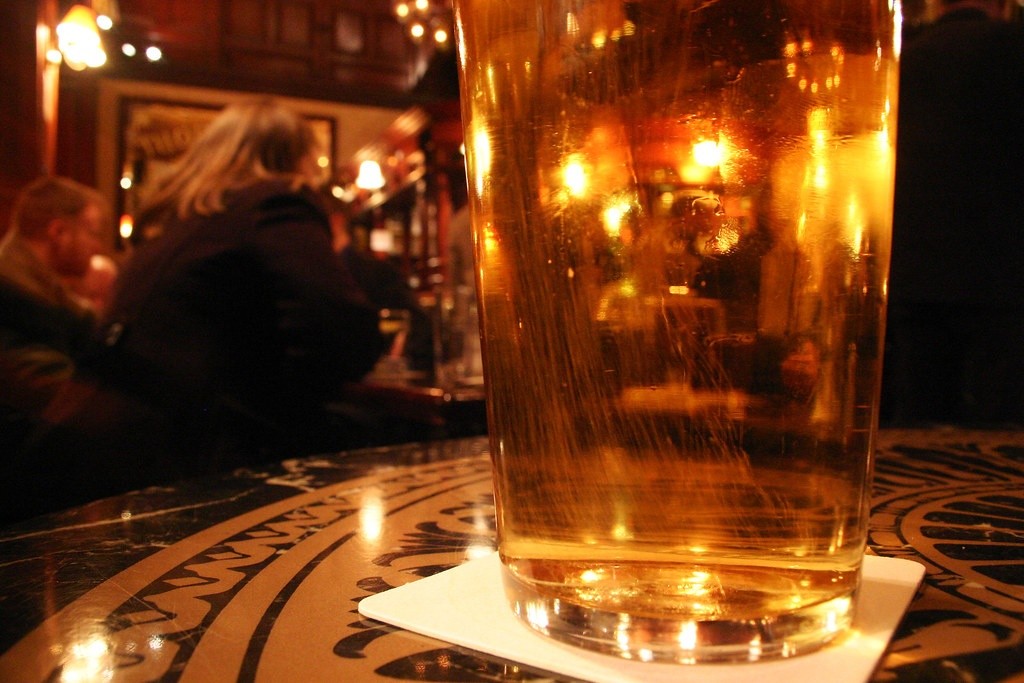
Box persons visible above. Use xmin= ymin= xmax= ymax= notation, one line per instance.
xmin=0 ymin=178 xmax=164 ymax=519
xmin=318 ymin=189 xmax=477 ymax=428
xmin=876 ymin=0 xmax=1024 ymax=428
xmin=87 ymin=98 xmax=440 ymax=486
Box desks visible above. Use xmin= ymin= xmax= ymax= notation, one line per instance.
xmin=0 ymin=426 xmax=1024 ymax=683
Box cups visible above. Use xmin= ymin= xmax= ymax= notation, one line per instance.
xmin=455 ymin=0 xmax=902 ymax=666
